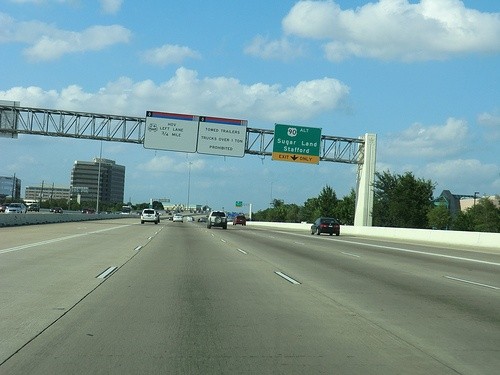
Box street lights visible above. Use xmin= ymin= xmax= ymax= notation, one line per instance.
xmin=474 ymin=191 xmax=480 ymax=205
xmin=187 ymin=162 xmax=192 ymax=213
xmin=95 ymin=121 xmax=107 ymax=214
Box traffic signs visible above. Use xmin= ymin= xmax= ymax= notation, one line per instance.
xmin=271 ymin=123 xmax=323 ymax=166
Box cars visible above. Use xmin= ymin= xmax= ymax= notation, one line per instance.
xmin=187 ymin=217 xmax=194 ymax=221
xmin=50 ymin=207 xmax=63 ymax=214
xmin=196 ymin=217 xmax=206 ymax=223
xmin=27 ymin=204 xmax=39 ymax=212
xmin=0 ymin=203 xmax=10 ymax=213
xmin=232 ymin=215 xmax=246 ymax=226
xmin=81 ymin=208 xmax=121 ymax=215
xmin=311 ymin=217 xmax=340 ymax=236
xmin=4 ymin=203 xmax=27 ymax=214
xmin=169 ymin=216 xmax=172 ymax=221
xmin=172 ymin=213 xmax=184 ymax=223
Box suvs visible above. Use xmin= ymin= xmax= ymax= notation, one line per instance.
xmin=140 ymin=209 xmax=160 ymax=224
xmin=206 ymin=211 xmax=229 ymax=229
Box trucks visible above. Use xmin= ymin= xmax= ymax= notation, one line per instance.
xmin=119 ymin=206 xmax=133 ymax=215
